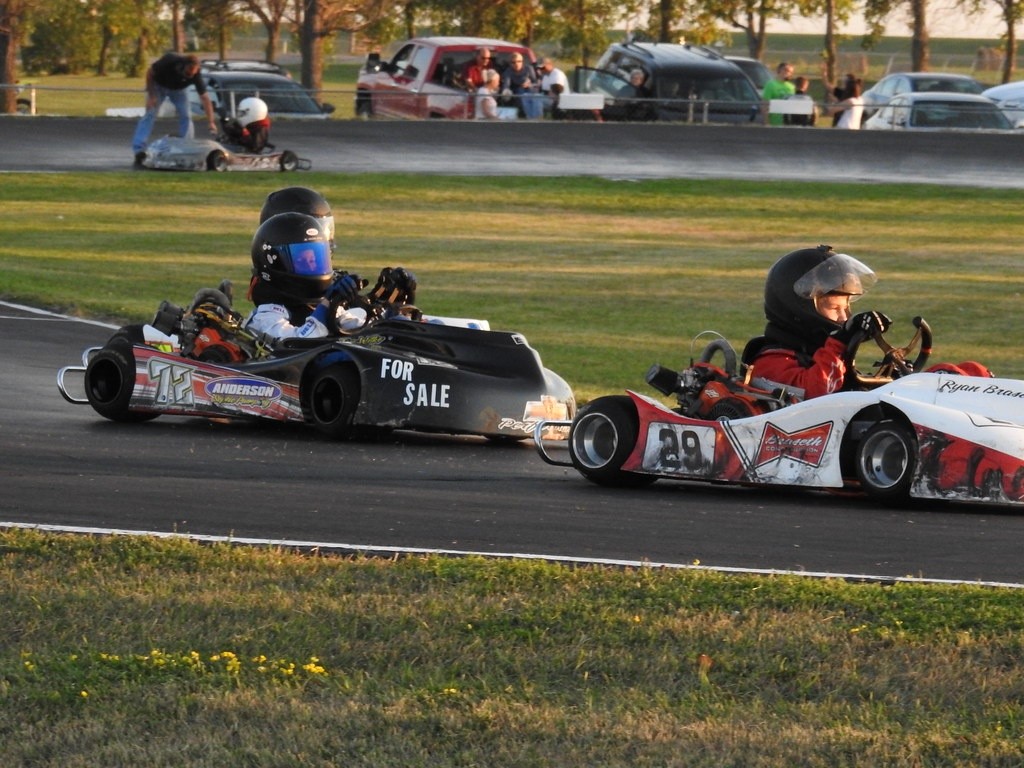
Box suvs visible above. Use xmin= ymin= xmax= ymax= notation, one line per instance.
xmin=569 ymin=40 xmax=777 ymax=125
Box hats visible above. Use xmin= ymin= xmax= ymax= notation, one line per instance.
xmin=535 ymin=58 xmax=550 ymax=69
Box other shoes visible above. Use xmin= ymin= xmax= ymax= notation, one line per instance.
xmin=133 ymin=152 xmax=146 ymax=169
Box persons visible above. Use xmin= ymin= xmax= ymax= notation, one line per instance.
xmin=820 ymin=61 xmax=865 ymax=129
xmin=218 ymin=97 xmax=271 ymax=155
xmin=615 ymin=68 xmax=650 ymax=105
xmin=760 ymin=63 xmax=797 ymax=125
xmin=245 ymin=187 xmax=415 ymax=342
xmin=783 ymin=77 xmax=818 ymax=126
xmin=465 ymin=48 xmax=570 ymax=120
xmin=739 ymin=245 xmax=992 ymax=404
xmin=133 ymin=52 xmax=218 ymax=165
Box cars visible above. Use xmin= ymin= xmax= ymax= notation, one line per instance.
xmin=186 ymin=58 xmax=338 ymax=119
xmin=859 ymin=91 xmax=1016 ymax=132
xmin=977 ymin=80 xmax=1024 ymax=131
xmin=860 ymin=70 xmax=985 ymax=125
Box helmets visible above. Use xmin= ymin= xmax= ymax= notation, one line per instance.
xmin=260 ymin=188 xmax=335 ymax=261
xmin=763 ymin=244 xmax=863 ymax=347
xmin=235 ymin=98 xmax=267 ymax=127
xmin=250 ymin=212 xmax=334 ymax=298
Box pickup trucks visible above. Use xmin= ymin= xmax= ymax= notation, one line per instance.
xmin=355 ymin=35 xmax=607 ymax=123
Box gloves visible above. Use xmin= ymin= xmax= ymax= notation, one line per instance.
xmin=829 ymin=310 xmax=893 ymax=346
xmin=224 ymin=122 xmax=242 ymax=137
xmin=324 ymin=274 xmax=370 ymax=309
xmin=371 ymin=265 xmax=416 ymax=306
